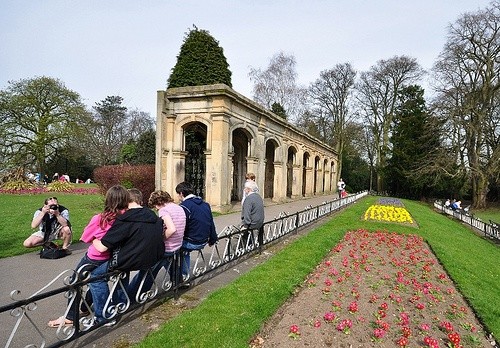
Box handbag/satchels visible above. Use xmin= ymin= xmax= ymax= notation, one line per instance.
xmin=40 ymin=250 xmax=68 ymax=259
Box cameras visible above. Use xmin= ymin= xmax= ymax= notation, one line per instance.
xmin=49 ymin=204 xmax=58 ymax=210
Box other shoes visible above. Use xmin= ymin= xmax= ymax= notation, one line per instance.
xmin=62 ymin=249 xmax=72 ymax=254
xmin=39 ymin=242 xmax=57 ymax=255
xmin=48 ymin=316 xmax=73 ymax=327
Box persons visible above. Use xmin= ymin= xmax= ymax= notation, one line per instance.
xmin=76 ymin=177 xmax=93 ymax=184
xmin=242 ymin=173 xmax=255 ymax=205
xmin=444 ymin=198 xmax=470 ymax=217
xmin=26 ymin=170 xmax=71 ymax=187
xmin=230 ymin=182 xmax=264 ymax=256
xmin=23 ymin=196 xmax=73 ymax=255
xmin=337 ymin=177 xmax=348 ymax=198
xmin=48 ymin=186 xmax=130 ymax=328
xmin=164 ymin=182 xmax=217 ymax=290
xmin=128 ymin=190 xmax=186 ymax=304
xmin=83 ymin=188 xmax=166 ymax=328
